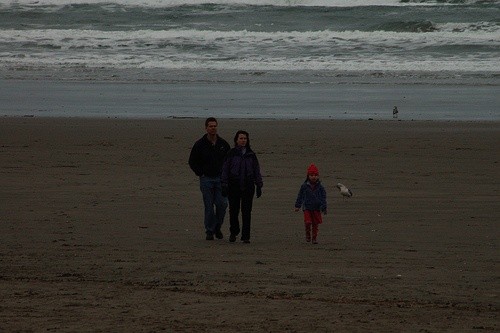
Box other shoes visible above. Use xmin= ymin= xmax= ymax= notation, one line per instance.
xmin=229 ymin=234 xmax=236 ymax=242
xmin=312 ymin=238 xmax=318 ymax=243
xmin=206 ymin=235 xmax=213 ymax=240
xmin=215 ymin=230 xmax=223 ymax=239
xmin=306 ymin=234 xmax=311 ymax=241
xmin=244 ymin=240 xmax=249 ymax=243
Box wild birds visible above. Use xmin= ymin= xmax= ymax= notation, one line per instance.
xmin=392 ymin=105 xmax=400 ymax=118
xmin=335 ymin=182 xmax=353 ymax=201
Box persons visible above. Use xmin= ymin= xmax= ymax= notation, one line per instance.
xmin=189 ymin=118 xmax=231 ymax=240
xmin=294 ymin=164 xmax=328 ymax=245
xmin=223 ymin=130 xmax=264 ymax=242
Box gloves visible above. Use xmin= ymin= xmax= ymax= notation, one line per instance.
xmin=221 ymin=185 xmax=228 ymax=196
xmin=256 ymin=186 xmax=261 ymax=198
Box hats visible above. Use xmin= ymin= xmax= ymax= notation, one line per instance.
xmin=308 ymin=165 xmax=318 ymax=175
